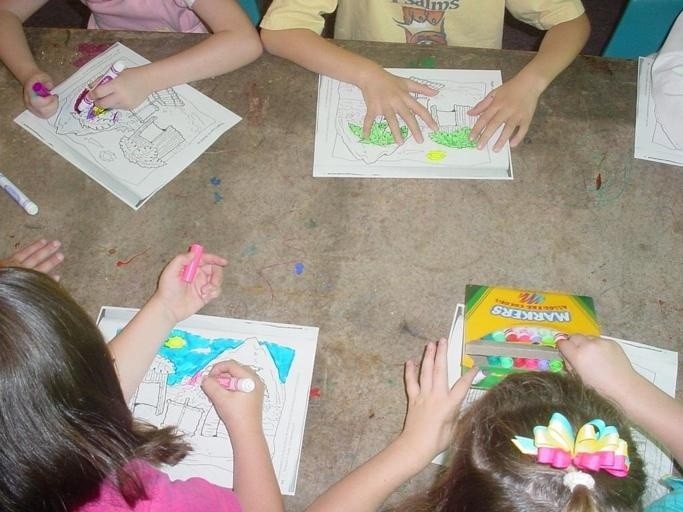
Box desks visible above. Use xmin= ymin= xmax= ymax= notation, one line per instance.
xmin=0 ymin=26 xmax=683 ymax=512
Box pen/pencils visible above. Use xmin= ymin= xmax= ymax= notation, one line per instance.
xmin=483 ymin=326 xmax=570 ymax=373
xmin=196 ymin=376 xmax=255 ymax=393
xmin=76 ymin=60 xmax=126 ymax=114
xmin=0 ymin=172 xmax=38 ymax=215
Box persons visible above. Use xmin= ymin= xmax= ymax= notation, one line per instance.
xmin=0 ymin=249 xmax=287 ymax=512
xmin=305 ymin=332 xmax=683 ymax=510
xmin=0 ymin=237 xmax=64 ymax=282
xmin=258 ymin=0 xmax=593 ymax=156
xmin=0 ymin=0 xmax=263 ymax=118
